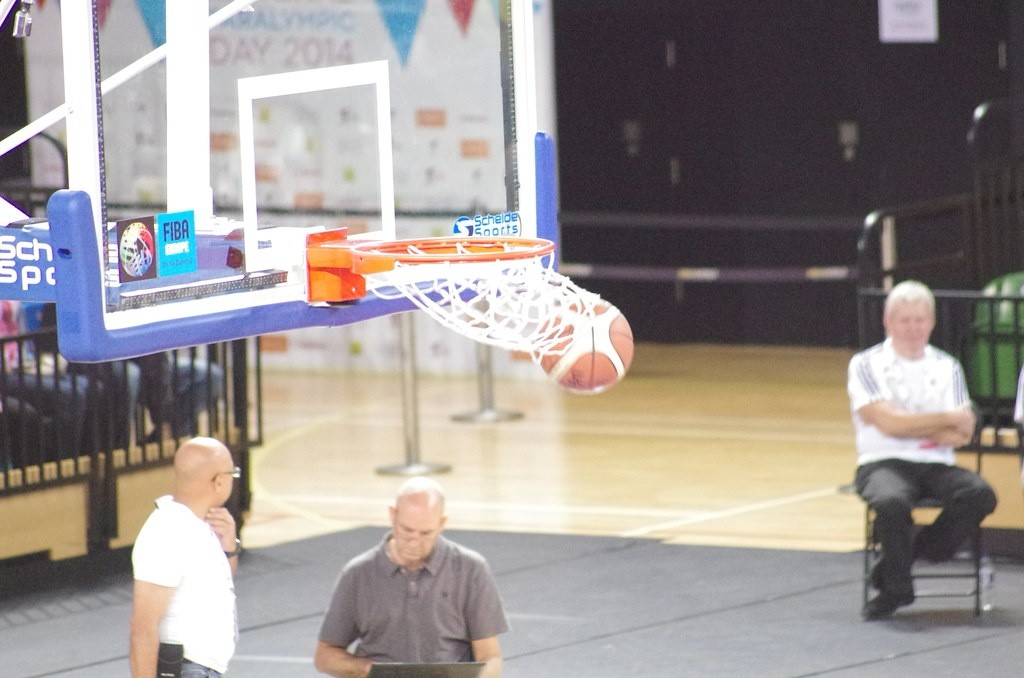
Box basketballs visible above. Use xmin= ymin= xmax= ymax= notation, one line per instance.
xmin=534 ymin=299 xmax=636 ymax=396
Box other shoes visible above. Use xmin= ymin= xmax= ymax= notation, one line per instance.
xmin=863 ymin=592 xmax=916 ymax=619
xmin=871 ymin=542 xmax=917 ymax=587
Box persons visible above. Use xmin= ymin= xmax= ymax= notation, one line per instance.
xmin=848 ymin=279 xmax=1002 ymax=620
xmin=312 ymin=476 xmax=510 ymax=678
xmin=130 ymin=436 xmax=242 ymax=678
xmin=0 ymin=299 xmax=226 ymax=472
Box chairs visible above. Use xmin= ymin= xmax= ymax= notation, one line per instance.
xmin=861 ymin=405 xmax=993 ymax=613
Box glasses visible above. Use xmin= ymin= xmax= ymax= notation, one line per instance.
xmin=394 ymin=512 xmax=441 ymax=542
xmin=211 ymin=466 xmax=242 ymax=482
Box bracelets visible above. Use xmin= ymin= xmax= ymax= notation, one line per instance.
xmin=224 ymin=539 xmax=242 ymax=558
